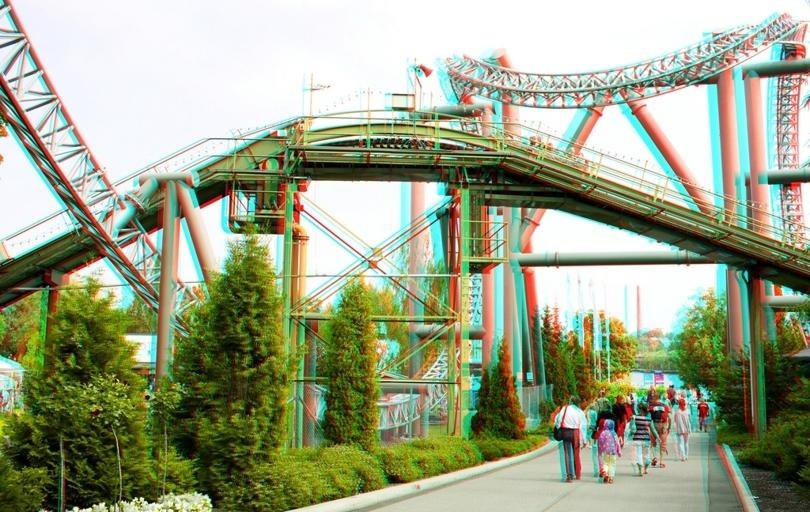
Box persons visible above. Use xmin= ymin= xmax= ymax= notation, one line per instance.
xmin=554 ymin=381 xmax=716 ymax=485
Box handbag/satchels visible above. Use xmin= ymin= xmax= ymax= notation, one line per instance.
xmin=630 ymin=414 xmax=636 ymax=433
xmin=553 ymin=425 xmax=564 ymax=441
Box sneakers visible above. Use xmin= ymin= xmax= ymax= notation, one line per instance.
xmin=650 ymin=456 xmax=658 ymax=467
xmin=680 ymin=457 xmax=688 ymax=463
xmin=642 ymin=470 xmax=649 ymax=474
xmin=586 ymin=444 xmax=592 ymax=449
xmin=561 ymin=476 xmax=574 ymax=483
xmin=598 ymin=466 xmax=615 ymax=485
xmin=631 ymin=473 xmax=644 ymax=477
xmin=659 ymin=463 xmax=666 ymax=468
